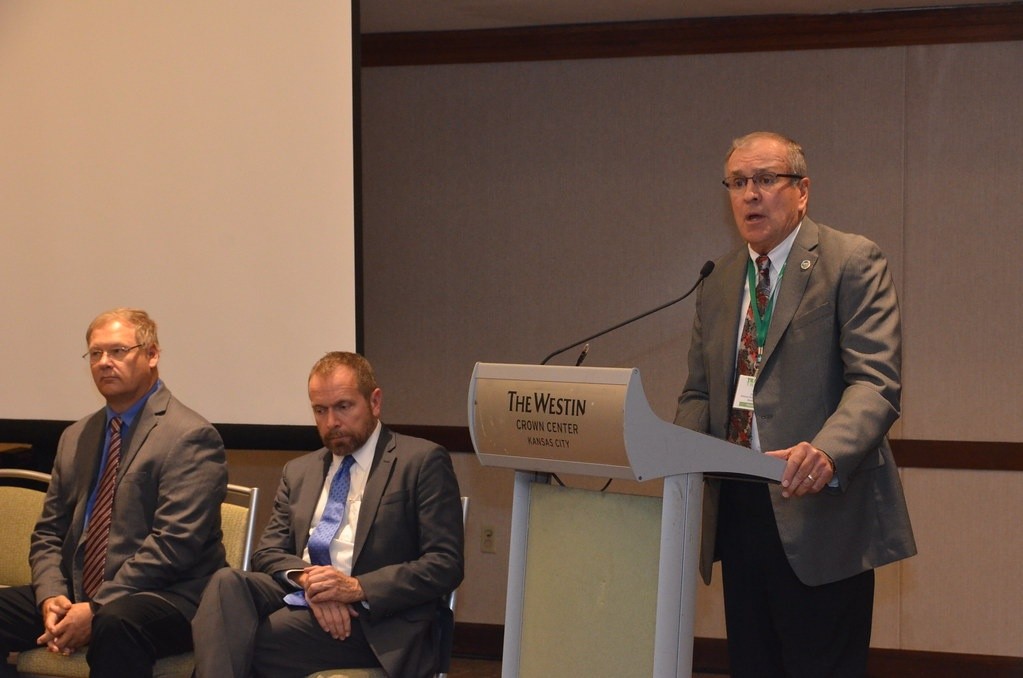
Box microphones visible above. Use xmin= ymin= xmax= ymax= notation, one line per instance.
xmin=541 ymin=260 xmax=715 ymax=365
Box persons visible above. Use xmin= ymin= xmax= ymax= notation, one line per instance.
xmin=0 ymin=308 xmax=231 ymax=678
xmin=671 ymin=131 xmax=918 ymax=678
xmin=191 ymin=351 xmax=466 ymax=678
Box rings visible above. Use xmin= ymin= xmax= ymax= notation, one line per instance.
xmin=808 ymin=474 xmax=815 ymax=482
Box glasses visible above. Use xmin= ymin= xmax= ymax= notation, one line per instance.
xmin=80 ymin=344 xmax=144 ymax=361
xmin=721 ymin=171 xmax=803 ymax=191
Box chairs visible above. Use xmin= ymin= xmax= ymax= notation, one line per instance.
xmin=310 ymin=495 xmax=469 ymax=678
xmin=17 ymin=484 xmax=260 ymax=678
xmin=0 ymin=468 xmax=52 ymax=588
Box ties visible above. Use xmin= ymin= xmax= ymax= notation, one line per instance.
xmin=282 ymin=453 xmax=356 ymax=609
xmin=80 ymin=416 xmax=125 ymax=603
xmin=726 ymin=255 xmax=771 ymax=449
